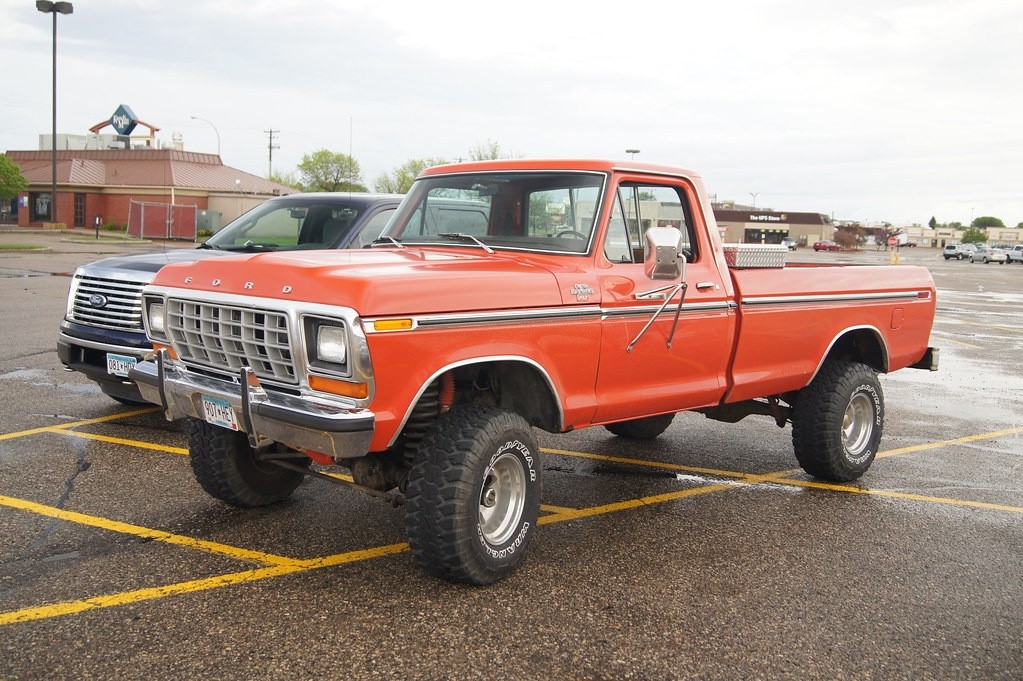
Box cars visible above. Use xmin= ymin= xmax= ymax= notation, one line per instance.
xmin=813 ymin=240 xmax=842 ymax=252
xmin=969 ymin=248 xmax=1008 ymax=265
xmin=943 ymin=245 xmax=974 ymax=260
xmin=899 ymin=242 xmax=917 ymax=248
xmin=781 ymin=237 xmax=798 ymax=251
xmin=1006 ymin=245 xmax=1023 ymax=264
xmin=962 ymin=243 xmax=1014 ymax=253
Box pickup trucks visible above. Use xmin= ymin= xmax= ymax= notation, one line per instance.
xmin=127 ymin=158 xmax=940 ymax=589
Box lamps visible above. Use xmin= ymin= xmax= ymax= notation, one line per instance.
xmin=94 ymin=214 xmax=104 ymax=226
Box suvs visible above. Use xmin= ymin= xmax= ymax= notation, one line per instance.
xmin=56 ymin=193 xmax=491 ymax=406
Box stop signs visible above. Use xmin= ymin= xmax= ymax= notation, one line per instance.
xmin=888 ymin=237 xmax=897 ymax=247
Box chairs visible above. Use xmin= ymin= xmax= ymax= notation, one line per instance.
xmin=320 ymin=217 xmax=363 ymax=249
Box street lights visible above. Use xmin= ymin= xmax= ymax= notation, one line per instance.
xmin=36 ymin=0 xmax=74 ymax=222
xmin=191 ymin=116 xmax=220 ymax=156
xmin=750 ymin=192 xmax=760 ymax=206
xmin=626 ymin=150 xmax=640 ymax=219
